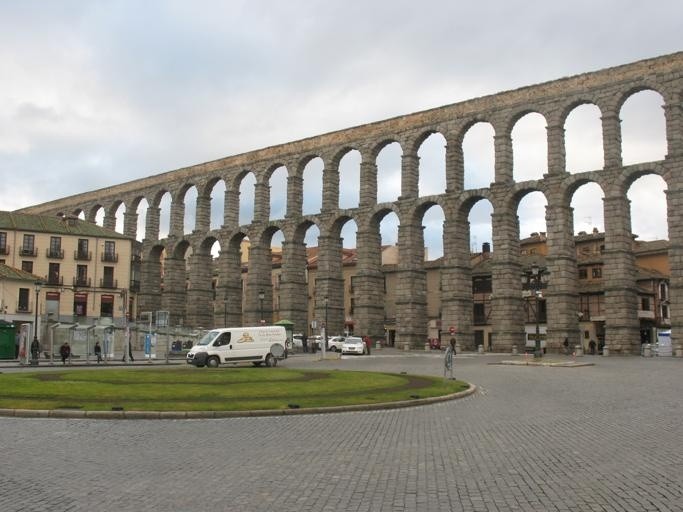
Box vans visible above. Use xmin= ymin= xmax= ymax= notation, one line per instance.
xmin=184 ymin=325 xmax=292 ymax=369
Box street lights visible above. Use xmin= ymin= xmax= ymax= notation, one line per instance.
xmin=223 ymin=297 xmax=229 ymax=329
xmin=30 ymin=277 xmax=42 ymax=365
xmin=518 ymin=263 xmax=550 ymax=359
xmin=323 ymin=295 xmax=329 ymax=347
xmin=257 ymin=288 xmax=266 ymax=324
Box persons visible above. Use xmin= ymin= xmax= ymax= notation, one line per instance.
xmin=95 ymin=341 xmax=102 ymax=364
xmin=15 ymin=331 xmax=21 ymax=359
xmin=123 ymin=336 xmax=135 ymax=362
xmin=362 ymin=335 xmax=372 ymax=356
xmin=588 ymin=339 xmax=596 ymax=354
xmin=31 ymin=335 xmax=41 ymax=359
xmin=443 ymin=344 xmax=453 ymax=377
xmin=643 ymin=332 xmax=650 ymax=343
xmin=562 ymin=337 xmax=569 ymax=355
xmin=300 ymin=333 xmax=309 ymax=353
xmin=450 ymin=336 xmax=457 ymax=355
xmin=59 ymin=342 xmax=70 ymax=365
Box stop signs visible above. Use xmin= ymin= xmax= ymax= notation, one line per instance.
xmin=449 ymin=327 xmax=454 ymax=333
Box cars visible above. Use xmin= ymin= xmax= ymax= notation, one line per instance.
xmin=292 ymin=334 xmax=349 ymax=352
xmin=340 ymin=336 xmax=367 ymax=355
